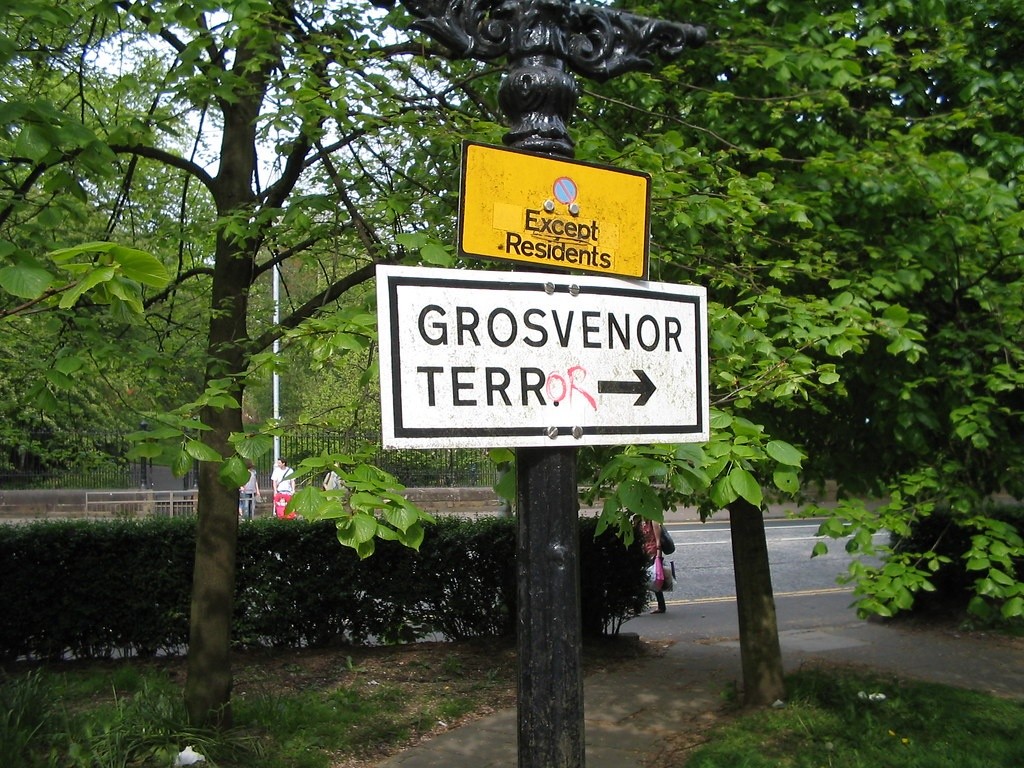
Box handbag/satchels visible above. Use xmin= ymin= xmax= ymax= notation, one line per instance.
xmin=644 ymin=558 xmax=677 ymax=593
xmin=650 ymin=518 xmax=675 ymax=555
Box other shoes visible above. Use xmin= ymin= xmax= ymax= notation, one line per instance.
xmin=650 ymin=609 xmax=665 ymax=614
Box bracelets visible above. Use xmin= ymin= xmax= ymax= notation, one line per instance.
xmin=656 ymin=546 xmax=663 ymax=550
xmin=656 ymin=556 xmax=662 ymax=558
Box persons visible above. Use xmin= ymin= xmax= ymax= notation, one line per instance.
xmin=642 ymin=520 xmax=666 ymax=614
xmin=239 ymin=464 xmax=260 ymax=521
xmin=271 ymin=458 xmax=295 ymax=497
xmin=323 ymin=464 xmax=348 ymax=501
